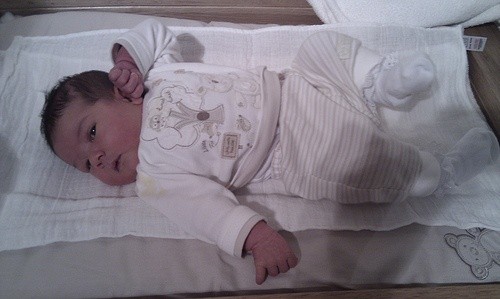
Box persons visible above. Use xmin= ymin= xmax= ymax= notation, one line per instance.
xmin=36 ymin=16 xmax=500 ymax=290
